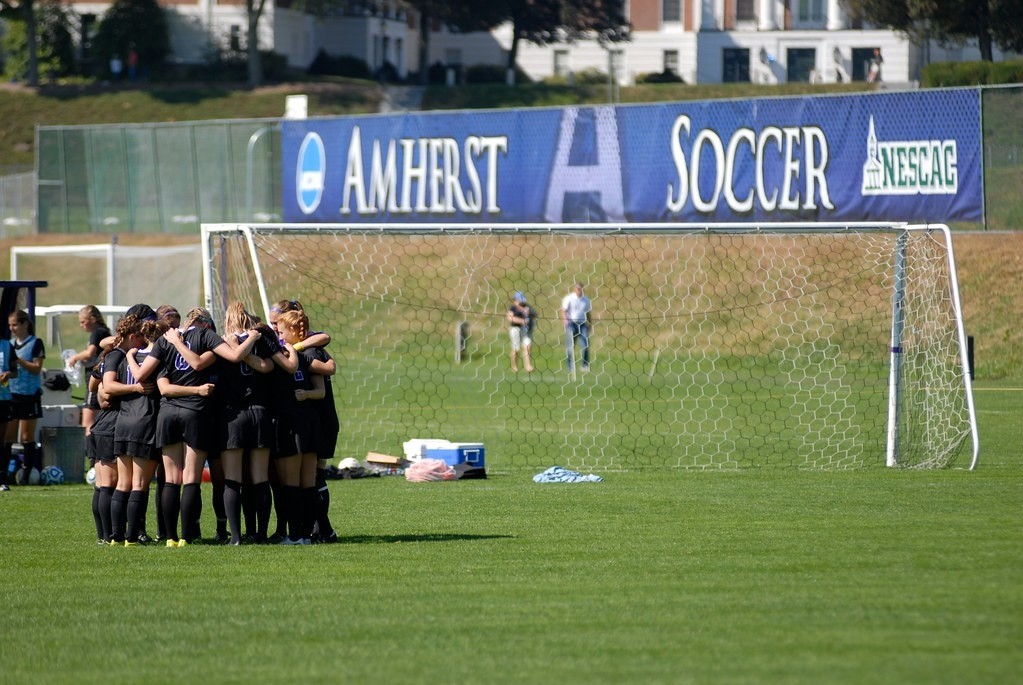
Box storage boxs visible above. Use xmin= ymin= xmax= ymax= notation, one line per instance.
xmin=402 ymin=438 xmax=451 ymax=463
xmin=427 ymin=442 xmax=485 ymax=468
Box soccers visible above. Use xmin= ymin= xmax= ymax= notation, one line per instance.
xmin=40 ymin=464 xmax=65 ymax=486
xmin=338 ymin=457 xmax=361 ymax=470
xmin=15 ymin=466 xmax=40 ymax=486
xmin=86 ymin=467 xmax=96 ymax=485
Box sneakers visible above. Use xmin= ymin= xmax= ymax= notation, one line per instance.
xmin=279 ymin=536 xmax=304 ymax=545
xmin=303 ymin=538 xmax=312 ymax=544
xmin=312 ymin=529 xmax=337 ymax=544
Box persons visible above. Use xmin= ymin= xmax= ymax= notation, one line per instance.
xmin=560 ymin=283 xmax=592 ymax=377
xmin=507 ymin=291 xmax=535 ymax=376
xmin=1 ymin=333 xmax=18 ymax=492
xmin=86 ymin=302 xmax=339 ymax=545
xmin=65 ymin=303 xmax=112 ymax=479
xmin=5 ymin=310 xmax=46 ymax=487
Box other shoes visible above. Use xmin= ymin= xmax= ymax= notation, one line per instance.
xmin=216 ymin=530 xmax=227 ymax=541
xmin=167 ymin=538 xmax=179 ymax=546
xmin=155 ymin=534 xmax=166 ymax=541
xmin=254 ymin=534 xmax=267 ymax=542
xmin=109 ymin=539 xmax=125 ymax=547
xmin=265 ymin=532 xmax=287 ymax=543
xmin=176 ymin=538 xmax=201 ymax=546
xmin=240 ymin=533 xmax=257 ymax=544
xmin=19 ymin=480 xmax=29 ymax=486
xmin=138 ymin=532 xmax=150 ymax=542
xmin=97 ymin=537 xmax=109 ymax=545
xmin=124 ymin=540 xmax=145 ymax=547
xmin=1 ymin=484 xmax=10 ymax=491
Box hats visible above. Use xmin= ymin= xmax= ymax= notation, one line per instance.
xmin=514 ymin=290 xmax=527 ymax=303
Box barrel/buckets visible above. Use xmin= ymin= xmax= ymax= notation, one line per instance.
xmin=41 ymin=427 xmax=87 ymax=485
